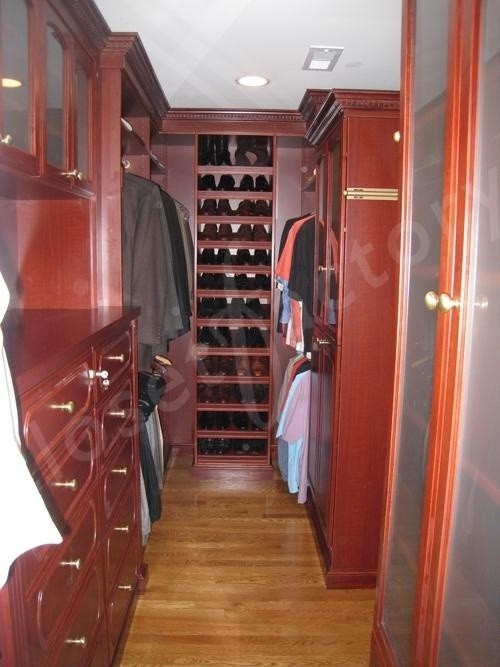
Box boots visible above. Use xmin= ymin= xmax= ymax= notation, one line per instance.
xmin=199 ymin=136 xmax=272 ymax=167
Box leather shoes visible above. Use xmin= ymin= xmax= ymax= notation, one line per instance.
xmin=195 ymin=327 xmax=267 ymax=405
xmin=197 ymin=197 xmax=272 ymax=319
xmin=255 ymin=175 xmax=270 ymax=192
xmin=218 ymin=175 xmax=235 ymax=191
xmin=197 ymin=413 xmax=265 ymax=457
xmin=239 ymin=174 xmax=253 ymax=192
xmin=201 ymin=175 xmax=216 ymax=192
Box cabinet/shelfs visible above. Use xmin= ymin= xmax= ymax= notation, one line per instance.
xmin=301 ymin=2 xmax=499 ymax=666
xmin=193 ymin=162 xmax=275 ymax=465
xmin=3 ymin=0 xmax=166 ymax=664
